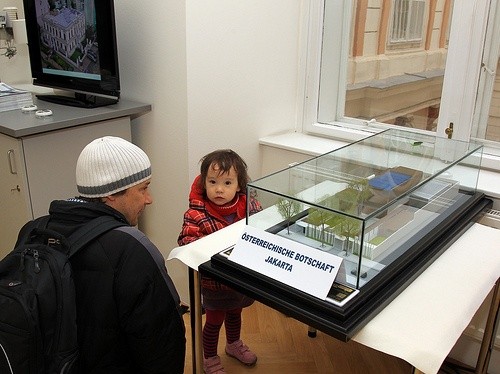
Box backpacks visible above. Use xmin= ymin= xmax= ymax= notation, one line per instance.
xmin=0 ymin=215 xmax=134 ymax=374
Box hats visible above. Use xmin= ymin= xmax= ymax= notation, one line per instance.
xmin=75 ymin=136 xmax=152 ymax=197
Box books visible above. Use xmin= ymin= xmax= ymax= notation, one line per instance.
xmin=0 ymin=83 xmax=34 ymax=111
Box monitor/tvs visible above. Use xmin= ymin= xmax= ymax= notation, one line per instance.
xmin=23 ymin=0 xmax=120 ymax=108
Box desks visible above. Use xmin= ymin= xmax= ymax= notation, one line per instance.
xmin=167 ymin=178 xmax=500 ymax=374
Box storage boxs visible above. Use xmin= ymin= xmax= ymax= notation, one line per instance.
xmin=198 ymin=127 xmax=493 ymax=342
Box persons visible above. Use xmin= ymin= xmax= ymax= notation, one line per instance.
xmin=15 ymin=136 xmax=187 ymax=374
xmin=177 ymin=149 xmax=263 ymax=374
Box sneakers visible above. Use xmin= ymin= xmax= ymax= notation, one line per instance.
xmin=224 ymin=340 xmax=257 ymax=365
xmin=203 ymin=355 xmax=226 ymax=374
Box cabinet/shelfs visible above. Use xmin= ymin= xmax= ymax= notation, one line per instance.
xmin=0 ymin=88 xmax=153 ymax=261
